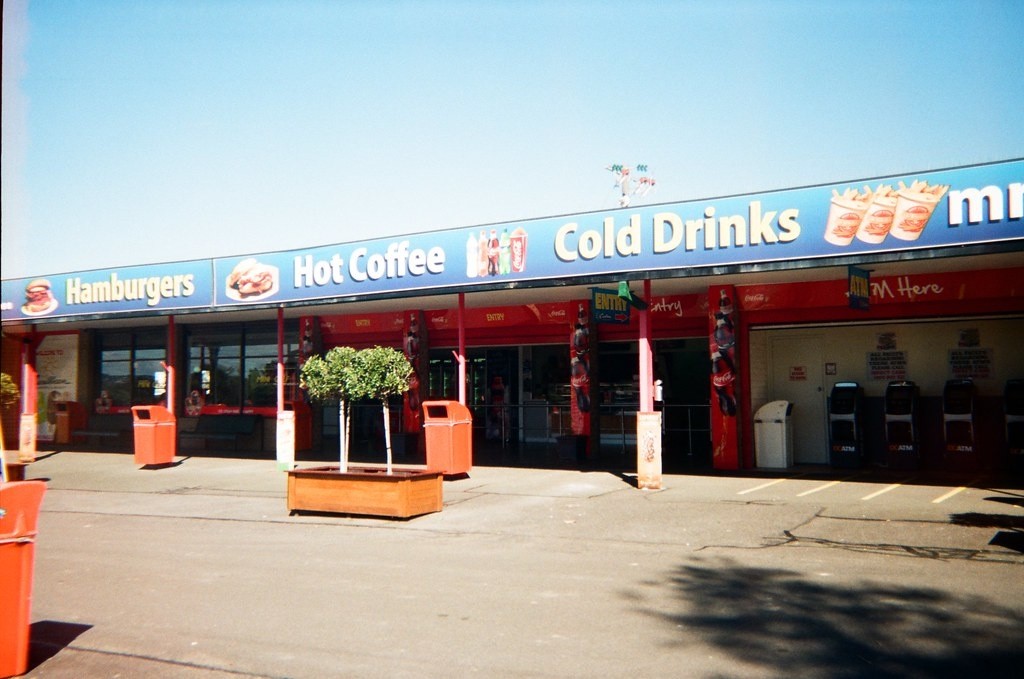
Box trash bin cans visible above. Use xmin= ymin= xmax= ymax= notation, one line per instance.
xmin=421 ymin=400 xmax=472 ymax=475
xmin=389 ymin=433 xmax=408 ymax=456
xmin=54 ymin=401 xmax=88 ymax=445
xmin=556 ymin=434 xmax=587 ymax=469
xmin=0 ymin=480 xmax=47 ymax=679
xmin=284 ymin=401 xmax=313 ymax=451
xmin=130 ymin=405 xmax=176 ymax=464
xmin=753 ymin=399 xmax=793 ymax=469
